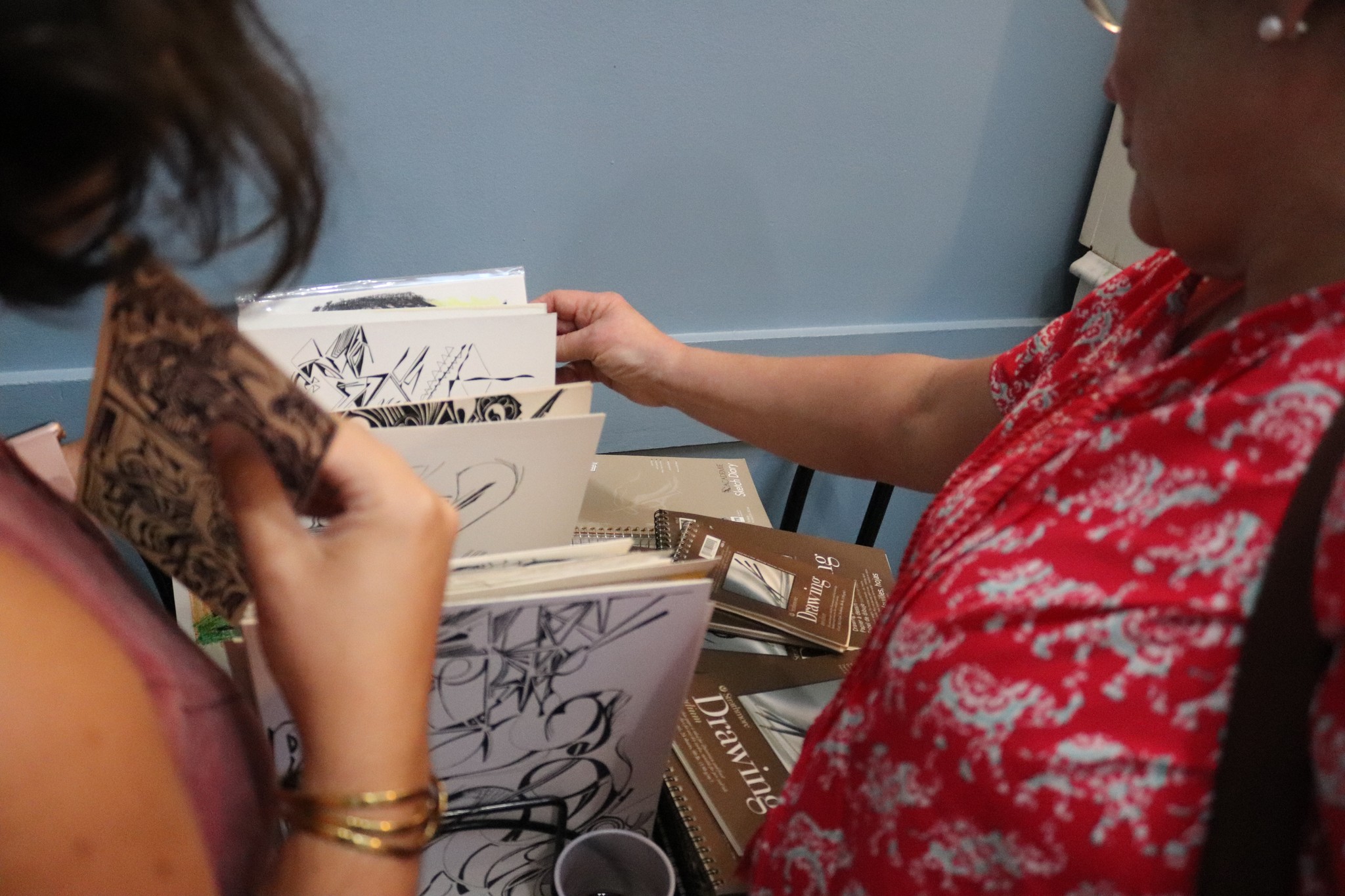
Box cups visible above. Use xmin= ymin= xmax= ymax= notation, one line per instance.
xmin=554 ymin=829 xmax=676 ymax=896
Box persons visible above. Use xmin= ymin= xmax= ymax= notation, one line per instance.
xmin=0 ymin=1 xmax=462 ymax=896
xmin=530 ymin=0 xmax=1345 ymax=896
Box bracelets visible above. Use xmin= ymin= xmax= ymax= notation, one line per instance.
xmin=277 ymin=776 xmax=449 ymax=857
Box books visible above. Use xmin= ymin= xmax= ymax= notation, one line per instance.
xmin=174 ymin=266 xmax=896 ymax=896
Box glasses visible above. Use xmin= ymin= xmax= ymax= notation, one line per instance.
xmin=1083 ymin=0 xmax=1123 ymax=33
